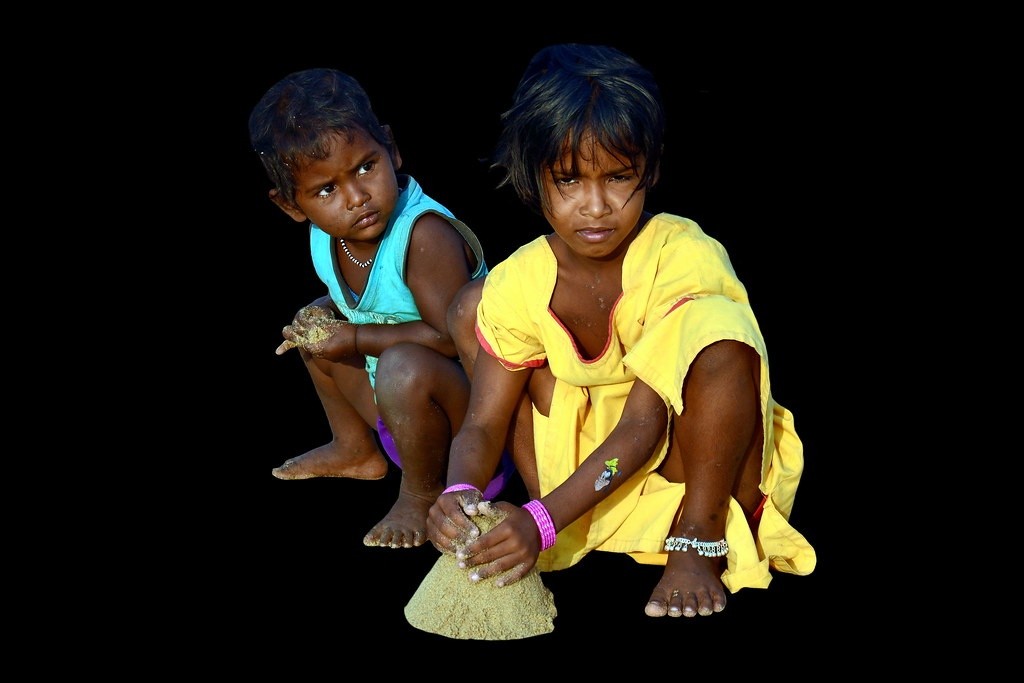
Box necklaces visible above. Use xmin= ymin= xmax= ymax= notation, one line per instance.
xmin=339 ymin=237 xmax=375 ymax=269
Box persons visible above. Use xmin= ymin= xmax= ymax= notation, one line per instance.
xmin=250 ymin=68 xmax=513 ymax=548
xmin=426 ymin=44 xmax=816 ymax=617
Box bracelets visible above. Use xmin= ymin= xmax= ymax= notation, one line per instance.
xmin=442 ymin=484 xmax=484 ymax=497
xmin=522 ymin=499 xmax=557 ymax=550
